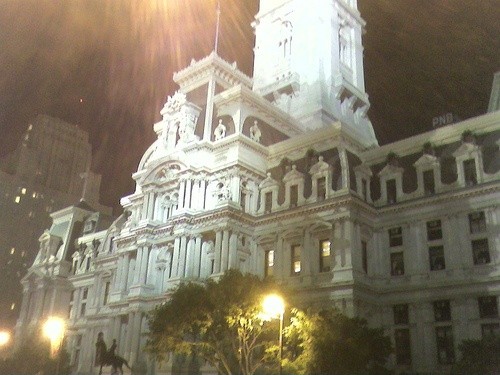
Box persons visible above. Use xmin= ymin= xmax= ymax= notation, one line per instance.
xmin=106 ymin=338 xmax=119 ymax=366
